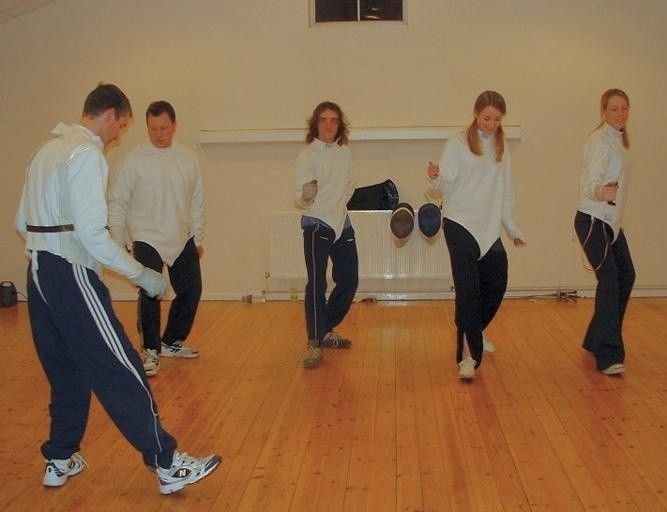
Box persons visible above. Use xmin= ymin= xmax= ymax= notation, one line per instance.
xmin=571 ymin=87 xmax=636 ymax=378
xmin=107 ymin=98 xmax=204 ymax=378
xmin=290 ymin=99 xmax=359 ymax=369
xmin=14 ymin=81 xmax=224 ymax=498
xmin=424 ymin=91 xmax=527 ymax=381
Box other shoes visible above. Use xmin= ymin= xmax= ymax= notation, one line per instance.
xmin=602 ymin=364 xmax=623 ymax=375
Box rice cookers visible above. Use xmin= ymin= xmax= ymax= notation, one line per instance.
xmin=0 ymin=281 xmax=17 ymax=306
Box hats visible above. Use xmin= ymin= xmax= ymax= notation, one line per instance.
xmin=391 ymin=203 xmax=415 ymax=240
xmin=418 ymin=204 xmax=441 ymax=238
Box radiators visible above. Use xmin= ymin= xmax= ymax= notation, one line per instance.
xmin=263 ymin=209 xmax=485 ymax=282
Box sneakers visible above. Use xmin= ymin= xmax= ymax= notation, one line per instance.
xmin=142 ymin=349 xmax=160 ymax=376
xmin=459 ymin=358 xmax=476 ymax=378
xmin=154 ymin=451 xmax=223 ymax=494
xmin=43 ymin=458 xmax=86 ymax=487
xmin=305 ymin=347 xmax=323 ymax=367
xmin=160 ymin=343 xmax=200 ymax=358
xmin=321 ymin=334 xmax=352 ymax=348
xmin=483 ymin=341 xmax=495 ymax=354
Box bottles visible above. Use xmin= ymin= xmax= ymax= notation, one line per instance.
xmin=241 ymin=295 xmax=267 ymax=304
xmin=290 ymin=287 xmax=298 ymax=304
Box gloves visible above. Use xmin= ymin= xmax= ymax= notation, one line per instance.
xmin=303 ymin=181 xmax=317 ymax=202
xmin=134 ymin=266 xmax=168 ymax=299
